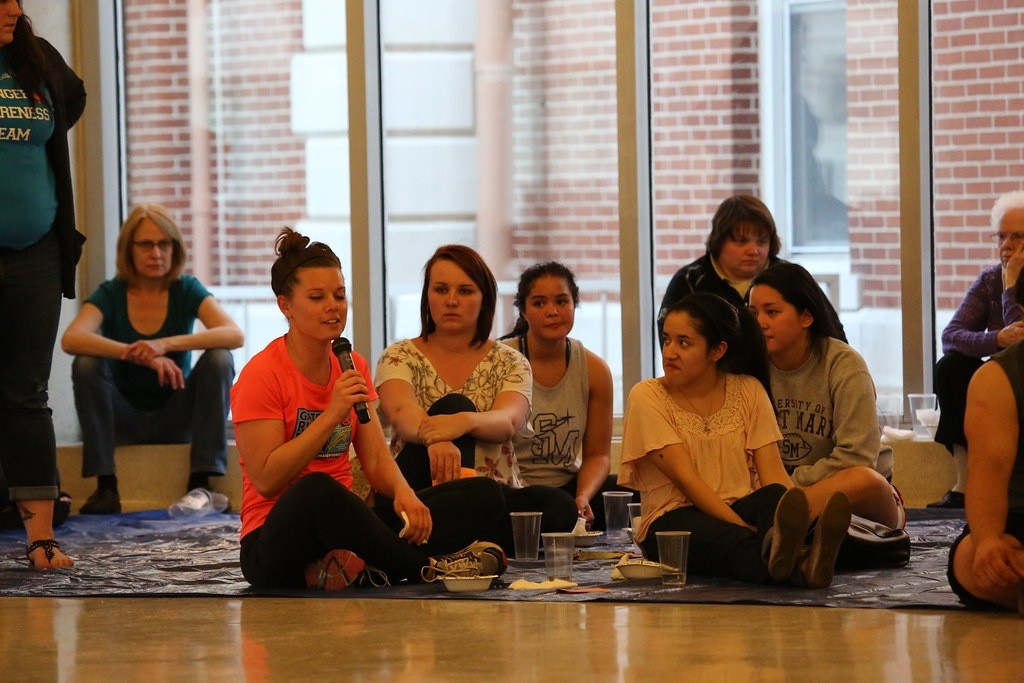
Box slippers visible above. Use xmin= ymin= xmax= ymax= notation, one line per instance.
xmin=27 ymin=539 xmax=60 ymax=562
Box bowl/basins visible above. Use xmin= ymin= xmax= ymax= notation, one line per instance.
xmin=438 ymin=575 xmax=498 ymax=594
xmin=542 ymin=533 xmax=603 ymax=547
xmin=923 ymin=425 xmax=938 ymax=439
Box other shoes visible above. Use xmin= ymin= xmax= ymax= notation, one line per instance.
xmin=926 ymin=490 xmax=964 ymax=513
xmin=800 ymin=490 xmax=851 ymax=588
xmin=768 ymin=488 xmax=810 ymax=582
xmin=80 ymin=489 xmax=121 ymax=514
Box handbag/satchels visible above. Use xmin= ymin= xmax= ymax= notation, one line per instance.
xmin=805 ymin=512 xmax=910 ymax=574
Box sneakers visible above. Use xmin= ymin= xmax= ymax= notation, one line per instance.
xmin=305 ymin=549 xmax=392 ymax=590
xmin=415 ymin=540 xmax=507 ymax=582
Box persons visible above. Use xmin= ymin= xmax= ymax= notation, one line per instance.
xmin=946 ymin=340 xmax=1024 ymax=613
xmin=62 ymin=204 xmax=244 ymax=516
xmin=748 ymin=263 xmax=907 ymax=532
xmin=498 ymin=261 xmax=641 ymax=531
xmin=0 ymin=0 xmax=87 ymax=570
xmin=374 ymin=244 xmax=579 ymax=548
xmin=657 ymin=195 xmax=850 ymax=348
xmin=942 ymin=191 xmax=1024 ymax=359
xmin=230 ymin=225 xmax=507 ymax=589
xmin=616 ymin=292 xmax=853 ymax=589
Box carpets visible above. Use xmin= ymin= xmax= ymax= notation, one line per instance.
xmin=0 ymin=509 xmax=969 ymax=610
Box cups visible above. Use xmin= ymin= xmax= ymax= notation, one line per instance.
xmin=510 ymin=512 xmax=544 ymax=561
xmin=908 ymin=393 xmax=936 ymax=438
xmin=540 ymin=533 xmax=577 ymax=582
xmin=655 ymin=531 xmax=690 ymax=584
xmin=627 ymin=502 xmax=642 ymax=556
xmin=602 ymin=490 xmax=633 ymax=540
xmin=875 ymin=397 xmax=901 ymax=433
xmin=168 ymin=490 xmax=210 ymax=521
xmin=195 ymin=487 xmax=229 ymax=515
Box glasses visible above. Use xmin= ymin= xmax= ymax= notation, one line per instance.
xmin=990 ymin=232 xmax=1024 ymax=242
xmin=133 ymin=240 xmax=174 ymax=248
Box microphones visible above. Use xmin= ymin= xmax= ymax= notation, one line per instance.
xmin=332 ymin=337 xmax=371 ymax=424
